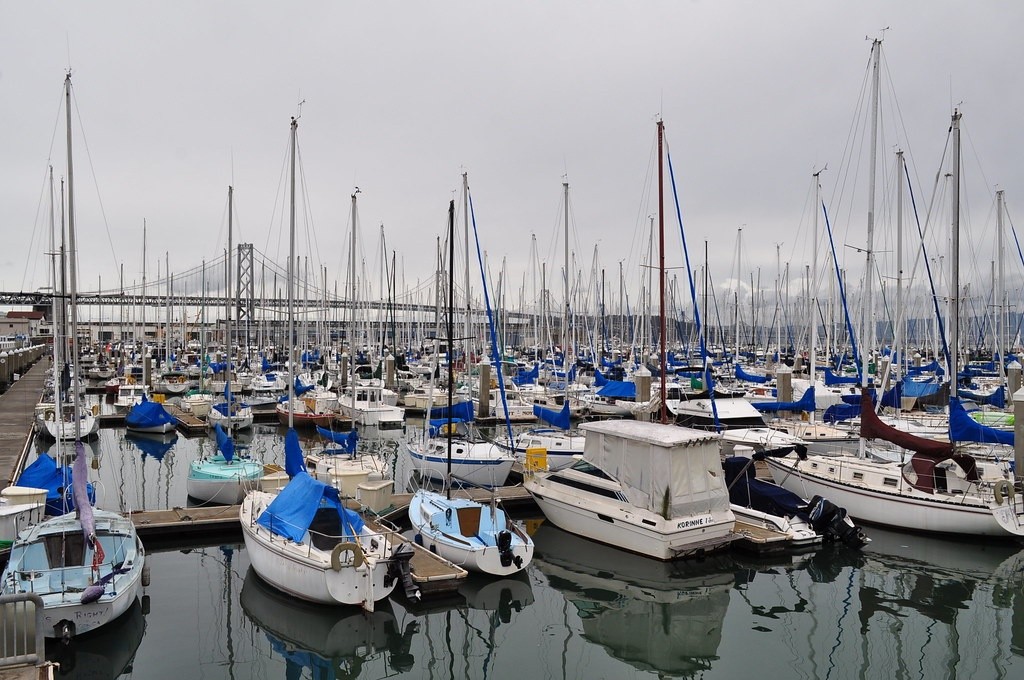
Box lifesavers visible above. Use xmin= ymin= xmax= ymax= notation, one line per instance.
xmin=993 ymin=480 xmax=1015 ymax=504
xmin=330 ymin=542 xmax=365 ymax=571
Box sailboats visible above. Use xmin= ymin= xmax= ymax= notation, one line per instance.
xmin=0 ymin=37 xmax=1023 ymax=645
xmin=40 ymin=521 xmax=1023 ymax=680
